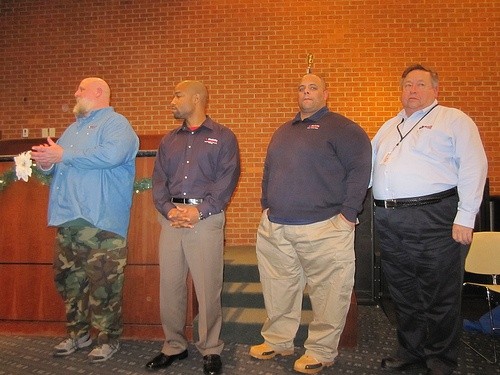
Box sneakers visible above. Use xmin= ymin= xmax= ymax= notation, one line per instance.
xmin=53 ymin=329 xmax=92 ymax=355
xmin=88 ymin=339 xmax=120 ymax=362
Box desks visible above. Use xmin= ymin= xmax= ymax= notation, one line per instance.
xmin=0 ymin=149 xmax=199 ymax=343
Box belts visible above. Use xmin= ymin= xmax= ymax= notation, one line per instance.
xmin=374 ymin=187 xmax=457 ymax=209
xmin=169 ymin=197 xmax=204 ymax=206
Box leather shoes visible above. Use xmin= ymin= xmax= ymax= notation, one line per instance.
xmin=144 ymin=347 xmax=189 ymax=371
xmin=382 ymin=354 xmax=424 ymax=373
xmin=427 ymin=367 xmax=455 ymax=375
xmin=202 ymin=353 xmax=222 ymax=375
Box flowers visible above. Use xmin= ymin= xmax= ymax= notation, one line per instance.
xmin=0 ymin=150 xmax=153 ymax=193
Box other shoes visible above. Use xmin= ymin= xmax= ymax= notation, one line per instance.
xmin=294 ymin=353 xmax=335 ymax=374
xmin=250 ymin=341 xmax=295 ymax=361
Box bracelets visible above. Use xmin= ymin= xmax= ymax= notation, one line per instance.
xmin=198 ymin=211 xmax=203 ymax=220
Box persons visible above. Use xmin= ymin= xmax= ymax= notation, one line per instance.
xmin=30 ymin=78 xmax=140 ymax=363
xmin=368 ymin=64 xmax=488 ymax=375
xmin=249 ymin=74 xmax=372 ymax=375
xmin=145 ymin=79 xmax=241 ymax=375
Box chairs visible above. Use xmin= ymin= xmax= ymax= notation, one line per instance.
xmin=460 ymin=231 xmax=500 ymax=365
